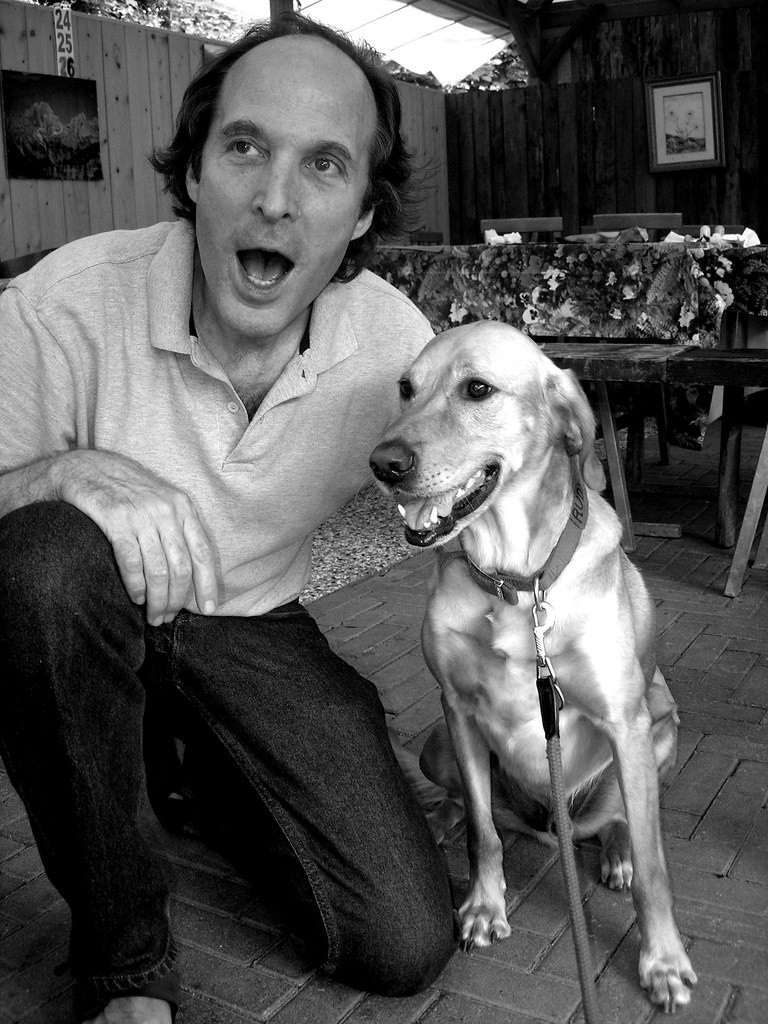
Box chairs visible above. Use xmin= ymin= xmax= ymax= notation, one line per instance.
xmin=409 ymin=230 xmax=444 ymax=246
xmin=593 ymin=214 xmax=682 ymax=241
xmin=480 ymin=218 xmax=563 ymax=242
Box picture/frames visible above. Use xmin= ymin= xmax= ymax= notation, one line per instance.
xmin=643 ymin=71 xmax=725 ymax=174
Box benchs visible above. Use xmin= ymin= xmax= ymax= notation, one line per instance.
xmin=533 ymin=343 xmax=768 ymax=598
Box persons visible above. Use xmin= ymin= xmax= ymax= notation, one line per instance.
xmin=0 ymin=21 xmax=459 ymax=1024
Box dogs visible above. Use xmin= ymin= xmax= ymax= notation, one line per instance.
xmin=369 ymin=319 xmax=699 ymax=1014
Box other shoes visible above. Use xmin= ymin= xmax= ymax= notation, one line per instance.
xmin=143 ymin=683 xmax=207 ymax=840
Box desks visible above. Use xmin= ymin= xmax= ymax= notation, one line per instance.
xmin=365 ymin=241 xmax=768 ymax=546
